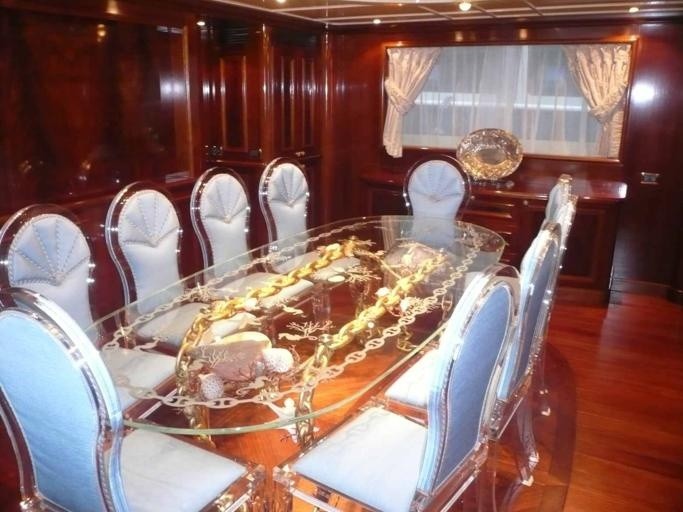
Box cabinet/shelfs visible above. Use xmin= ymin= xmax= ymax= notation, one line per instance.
xmin=354 ymin=154 xmax=629 ymax=308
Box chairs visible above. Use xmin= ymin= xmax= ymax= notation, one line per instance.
xmin=105 ymin=181 xmax=184 ymax=306
xmin=378 ymin=219 xmax=562 ymax=489
xmin=0 ymin=204 xmax=98 ymax=332
xmin=0 ymin=286 xmax=268 ymax=512
xmin=546 ymin=174 xmax=573 ymax=221
xmin=190 ymin=166 xmax=252 ymax=269
xmin=402 ymin=154 xmax=472 ymax=221
xmin=272 ymin=262 xmax=522 ymax=512
xmin=258 ymin=157 xmax=310 ymax=243
xmin=554 ymin=193 xmax=578 ymax=250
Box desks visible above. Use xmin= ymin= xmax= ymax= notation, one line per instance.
xmin=86 ymin=215 xmax=505 ymax=508
